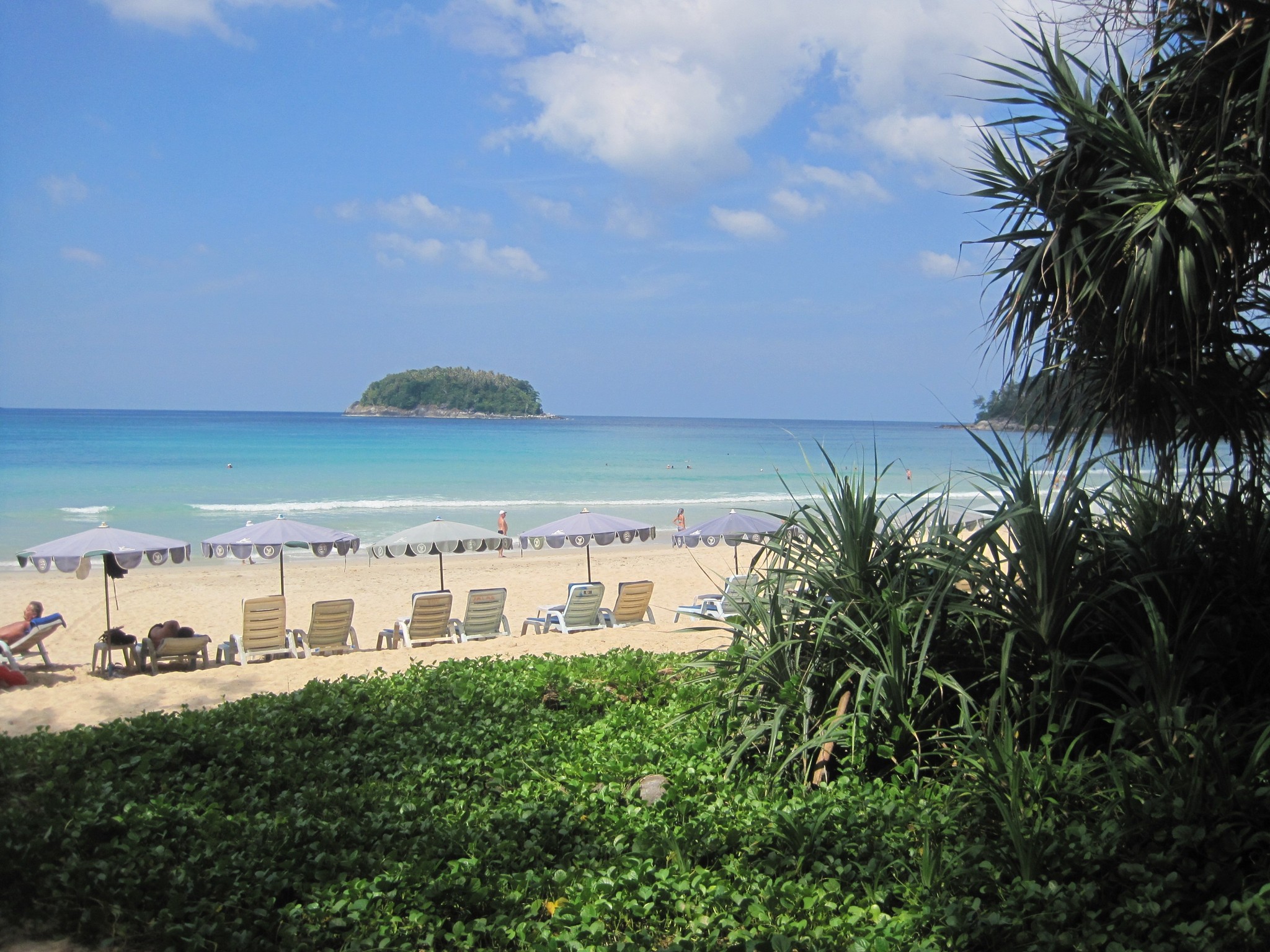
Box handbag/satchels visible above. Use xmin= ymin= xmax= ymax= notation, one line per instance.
xmin=98 ymin=626 xmax=138 ymax=647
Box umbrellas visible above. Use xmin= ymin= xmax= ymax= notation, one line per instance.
xmin=972 ymin=502 xmax=1013 ymax=549
xmin=367 ymin=516 xmax=513 ymax=589
xmin=895 ymin=503 xmax=990 ymax=537
xmin=518 ymin=507 xmax=656 ymax=582
xmin=15 ymin=520 xmax=191 ymax=662
xmin=200 ymin=514 xmax=360 ymax=593
xmin=672 ymin=507 xmax=798 ymax=574
xmin=796 ymin=513 xmax=922 ymax=544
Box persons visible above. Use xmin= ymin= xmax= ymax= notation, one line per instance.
xmin=498 ymin=510 xmax=508 ymax=557
xmin=673 ymin=508 xmax=686 ymax=532
xmin=667 ymin=464 xmax=674 ymax=468
xmin=147 ymin=621 xmax=194 ymax=645
xmin=687 ymin=465 xmax=691 ymax=468
xmin=907 ymin=469 xmax=911 ymax=479
xmin=0 ymin=601 xmax=43 ymax=654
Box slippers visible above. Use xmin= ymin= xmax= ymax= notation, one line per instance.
xmin=114 ymin=662 xmax=124 ymax=673
xmin=499 ymin=556 xmax=507 ymax=558
xmin=107 ymin=663 xmax=115 ymax=674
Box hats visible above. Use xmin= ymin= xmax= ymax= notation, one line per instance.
xmin=499 ymin=510 xmax=507 ymax=514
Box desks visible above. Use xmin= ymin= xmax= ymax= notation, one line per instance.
xmin=534 ymin=605 xmax=565 ymax=631
xmin=91 ymin=642 xmax=141 ymax=673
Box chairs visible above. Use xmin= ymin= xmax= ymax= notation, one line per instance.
xmin=521 ymin=580 xmax=656 ymax=635
xmin=376 ymin=588 xmax=511 ymax=651
xmin=129 ymin=634 xmax=212 ymax=675
xmin=0 ymin=613 xmax=66 ymax=671
xmin=674 ymin=567 xmax=800 ymax=622
xmin=216 ymin=595 xmax=358 ymax=666
